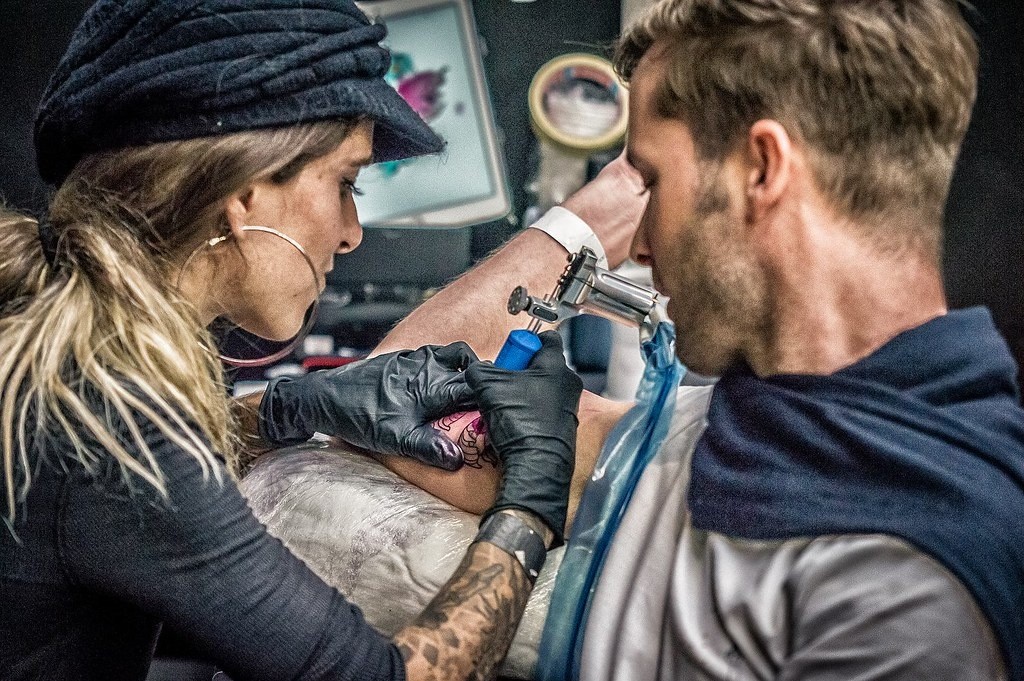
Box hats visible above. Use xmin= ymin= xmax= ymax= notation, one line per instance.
xmin=32 ymin=0 xmax=457 ymax=189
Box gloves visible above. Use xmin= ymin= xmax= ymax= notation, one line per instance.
xmin=460 ymin=330 xmax=585 ymax=554
xmin=253 ymin=336 xmax=498 ymax=471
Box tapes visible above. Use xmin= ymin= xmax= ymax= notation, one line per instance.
xmin=527 ymin=52 xmax=630 ymax=158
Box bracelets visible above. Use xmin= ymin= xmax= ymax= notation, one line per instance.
xmin=467 ymin=511 xmax=547 ymax=590
xmin=526 ymin=205 xmax=610 ymax=271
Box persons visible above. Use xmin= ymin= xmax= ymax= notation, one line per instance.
xmin=0 ymin=0 xmax=586 ymax=681
xmin=334 ymin=0 xmax=1024 ymax=680
